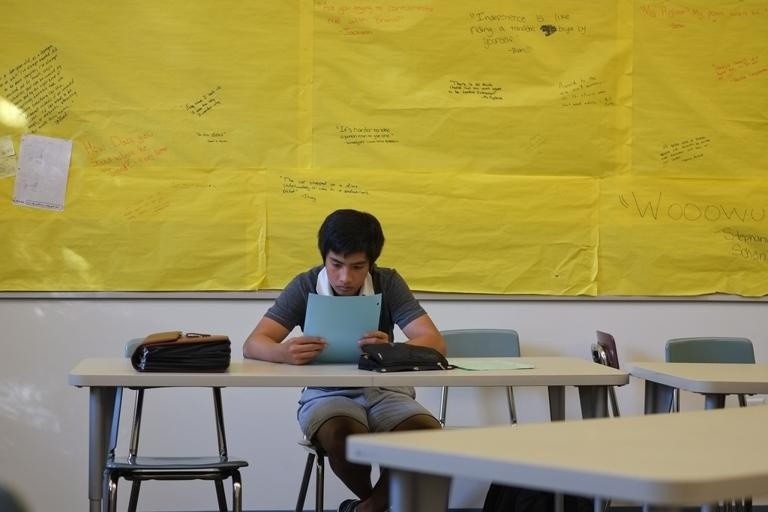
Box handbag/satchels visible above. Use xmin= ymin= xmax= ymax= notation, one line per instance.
xmin=132 ymin=332 xmax=231 ymax=373
xmin=358 ymin=343 xmax=448 ymax=373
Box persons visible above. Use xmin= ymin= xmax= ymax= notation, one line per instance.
xmin=243 ymin=209 xmax=447 ymax=511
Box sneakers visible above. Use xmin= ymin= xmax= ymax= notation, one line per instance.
xmin=338 ymin=499 xmax=359 ymax=511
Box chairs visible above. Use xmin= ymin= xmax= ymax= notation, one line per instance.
xmin=438 ymin=330 xmax=522 ymax=426
xmin=105 ymin=339 xmax=249 ymax=512
xmin=292 ymin=438 xmax=329 ymax=512
xmin=590 ymin=330 xmax=756 ymax=512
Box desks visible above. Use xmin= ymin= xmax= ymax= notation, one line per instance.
xmin=69 ymin=358 xmax=767 ymax=512
